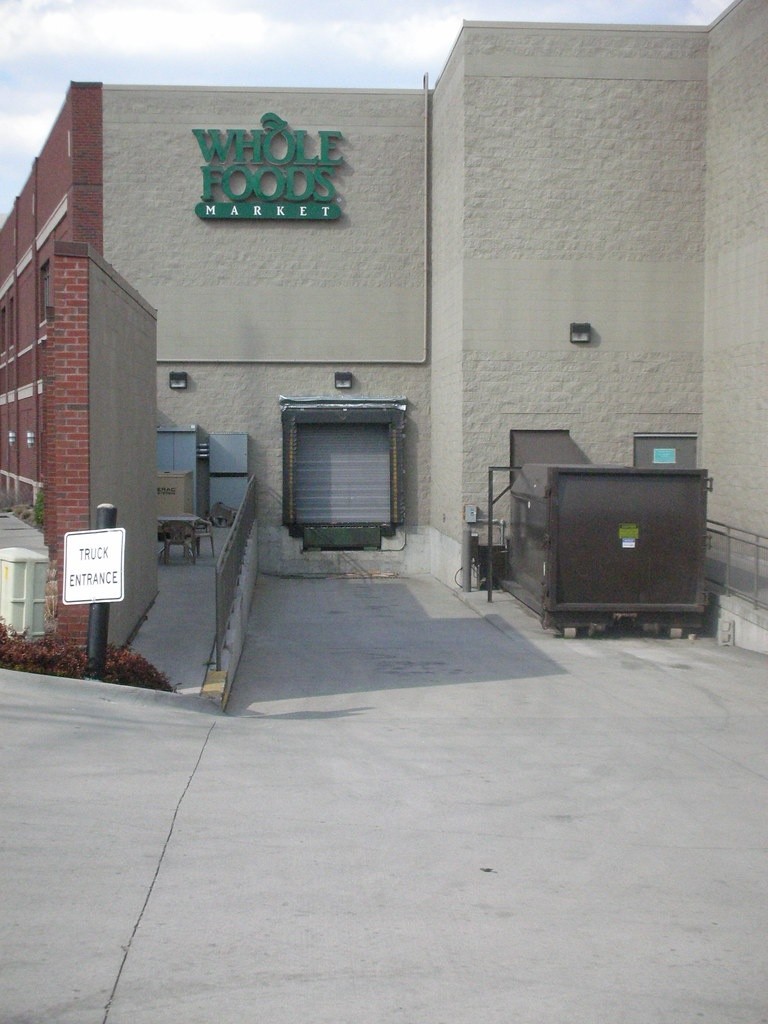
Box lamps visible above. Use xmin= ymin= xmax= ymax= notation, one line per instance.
xmin=570 ymin=323 xmax=591 ymax=343
xmin=335 ymin=372 xmax=352 ymax=388
xmin=169 ymin=369 xmax=189 ymax=389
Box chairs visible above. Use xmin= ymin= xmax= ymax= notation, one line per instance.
xmin=161 ymin=513 xmax=215 ymax=565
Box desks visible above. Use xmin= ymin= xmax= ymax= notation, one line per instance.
xmin=158 ymin=516 xmax=199 ymax=554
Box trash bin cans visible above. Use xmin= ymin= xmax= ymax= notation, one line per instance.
xmin=0 ymin=547 xmax=47 ymax=642
xmin=502 ymin=463 xmax=715 ymax=640
